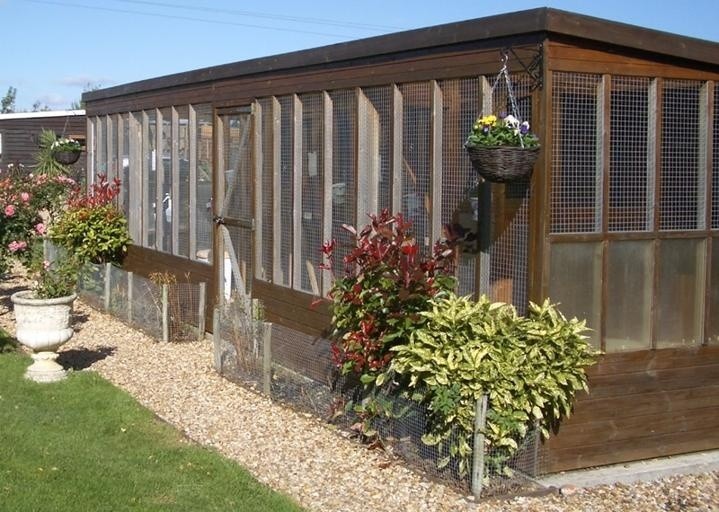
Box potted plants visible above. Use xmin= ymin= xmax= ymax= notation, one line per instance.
xmin=10 ymin=250 xmax=92 ymax=383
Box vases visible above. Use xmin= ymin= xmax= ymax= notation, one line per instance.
xmin=51 ymin=149 xmax=81 ymax=164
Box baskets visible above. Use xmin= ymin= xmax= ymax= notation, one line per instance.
xmin=54 ymin=150 xmax=80 ymax=164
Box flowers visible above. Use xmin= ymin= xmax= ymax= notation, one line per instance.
xmin=466 ymin=113 xmax=539 ymax=148
xmin=50 ymin=137 xmax=81 ymax=153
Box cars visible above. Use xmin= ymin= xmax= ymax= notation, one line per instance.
xmin=96 ymin=155 xmax=213 ymax=243
